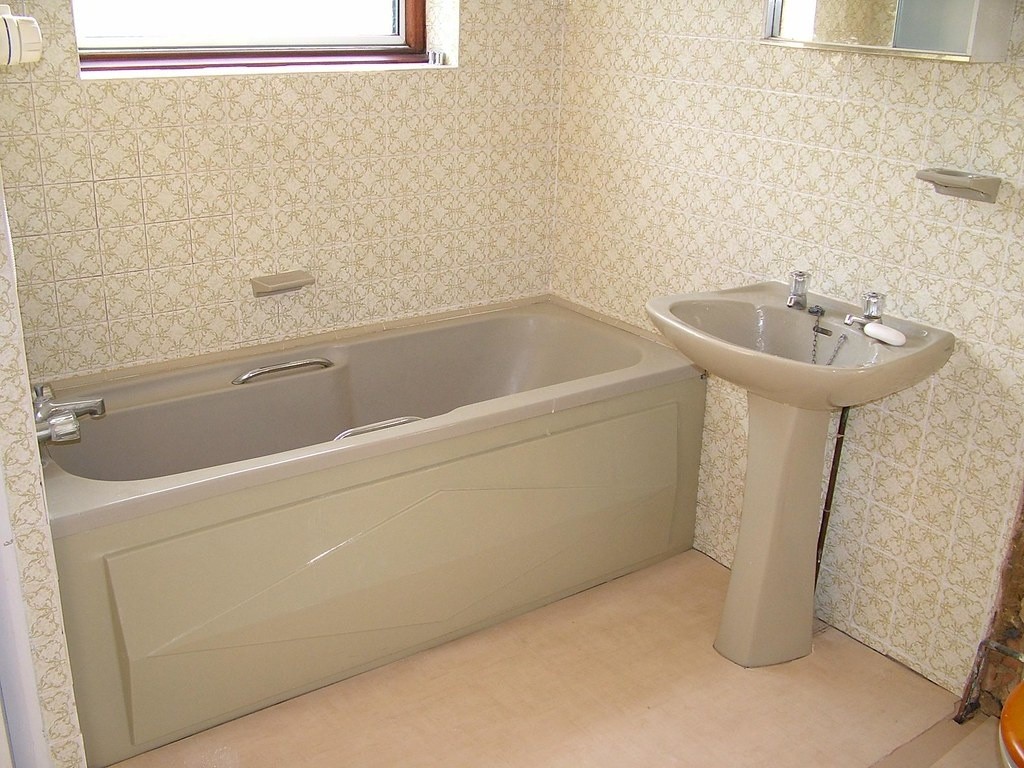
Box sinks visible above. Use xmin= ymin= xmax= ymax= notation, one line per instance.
xmin=645 ymin=276 xmax=957 ymax=411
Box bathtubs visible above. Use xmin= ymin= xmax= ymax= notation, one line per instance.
xmin=21 ymin=292 xmax=716 ymax=768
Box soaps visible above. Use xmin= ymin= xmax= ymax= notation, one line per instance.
xmin=861 ymin=322 xmax=907 ymax=347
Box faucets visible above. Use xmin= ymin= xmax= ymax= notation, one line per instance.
xmin=785 ymin=270 xmax=813 ymax=310
xmin=32 ymin=383 xmax=110 ymax=436
xmin=843 ymin=290 xmax=885 ymax=332
